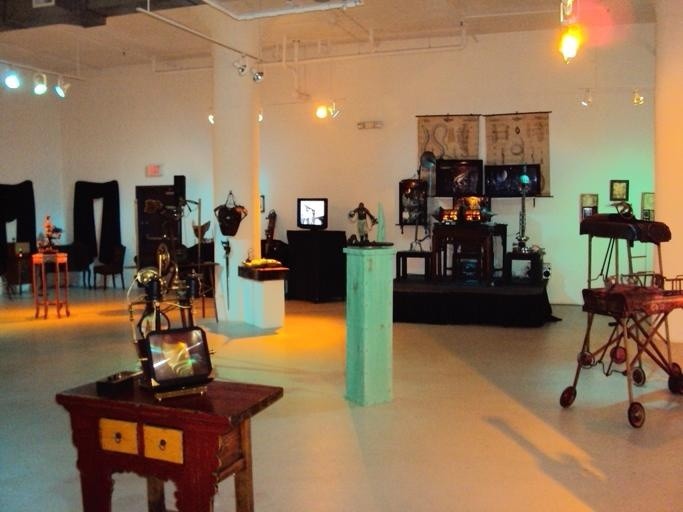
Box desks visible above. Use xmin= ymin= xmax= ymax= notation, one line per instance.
xmin=431 ymin=220 xmax=508 ymax=287
xmin=52 ymin=369 xmax=283 ymax=512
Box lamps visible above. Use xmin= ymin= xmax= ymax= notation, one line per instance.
xmin=577 ymin=85 xmax=645 ymax=109
xmin=230 ymin=48 xmax=264 ymax=84
xmin=314 ymin=100 xmax=342 ymax=122
xmin=556 ymin=0 xmax=585 ymax=65
xmin=0 ymin=34 xmax=86 ymax=100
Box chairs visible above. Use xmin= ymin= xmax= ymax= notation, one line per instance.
xmin=1 ymin=238 xmax=127 ymax=301
xmin=136 ymin=235 xmax=285 ymax=299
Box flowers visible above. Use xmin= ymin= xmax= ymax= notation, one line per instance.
xmin=35 ymin=212 xmax=63 ymax=250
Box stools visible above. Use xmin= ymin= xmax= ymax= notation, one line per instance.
xmin=27 ymin=251 xmax=69 ymax=320
xmin=394 ymin=249 xmax=434 ymax=284
xmin=502 ymin=249 xmax=544 ymax=283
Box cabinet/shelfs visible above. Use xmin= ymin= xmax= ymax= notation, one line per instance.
xmin=285 ymin=229 xmax=349 ymax=304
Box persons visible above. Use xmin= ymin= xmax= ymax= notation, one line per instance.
xmin=349 ymin=202 xmax=378 ymax=244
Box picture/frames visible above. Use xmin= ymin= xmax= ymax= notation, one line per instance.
xmin=609 ymin=178 xmax=629 ymax=202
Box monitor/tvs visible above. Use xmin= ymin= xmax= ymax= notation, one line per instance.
xmin=297 ymin=197 xmax=328 ymax=231
